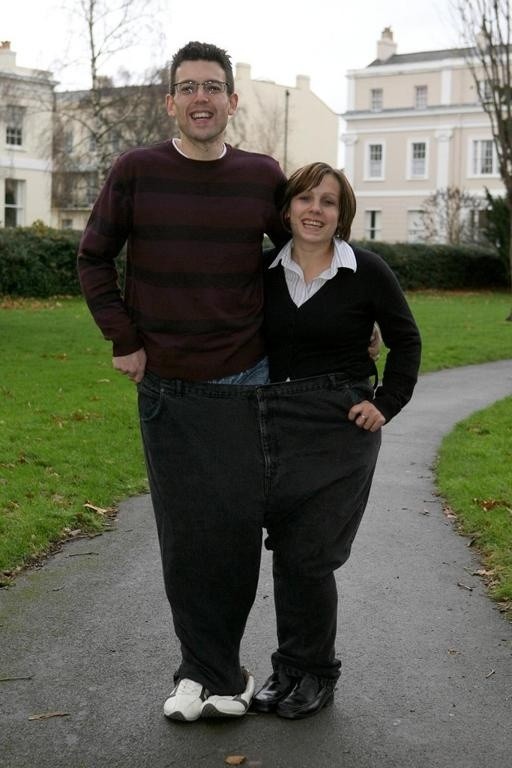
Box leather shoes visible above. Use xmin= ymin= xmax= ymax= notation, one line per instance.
xmin=252 ymin=662 xmax=336 ymax=720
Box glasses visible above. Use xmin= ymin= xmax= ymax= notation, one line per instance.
xmin=172 ymin=79 xmax=232 ymax=99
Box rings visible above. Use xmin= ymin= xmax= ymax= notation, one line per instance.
xmin=360 ymin=414 xmax=368 ymax=420
xmin=125 ymin=373 xmax=136 ymax=379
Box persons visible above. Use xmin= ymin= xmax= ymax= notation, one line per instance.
xmin=246 ymin=162 xmax=421 ymax=721
xmin=78 ymin=38 xmax=382 ymax=724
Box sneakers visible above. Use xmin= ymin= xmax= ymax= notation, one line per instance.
xmin=162 ymin=668 xmax=258 ymax=724
